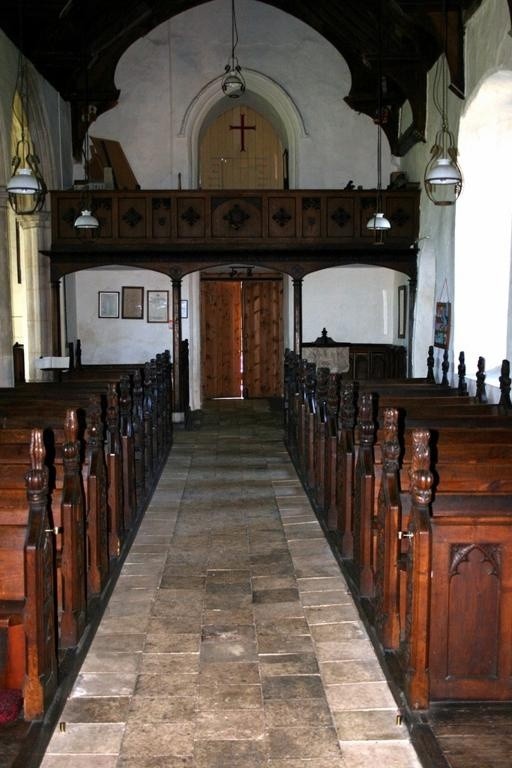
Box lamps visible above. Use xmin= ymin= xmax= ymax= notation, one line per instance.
xmin=219 ymin=0 xmax=247 ymax=100
xmin=364 ymin=50 xmax=463 ymax=247
xmin=6 ymin=53 xmax=102 ymax=247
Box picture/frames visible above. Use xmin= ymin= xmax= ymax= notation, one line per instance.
xmin=98 ymin=286 xmax=170 ymax=324
